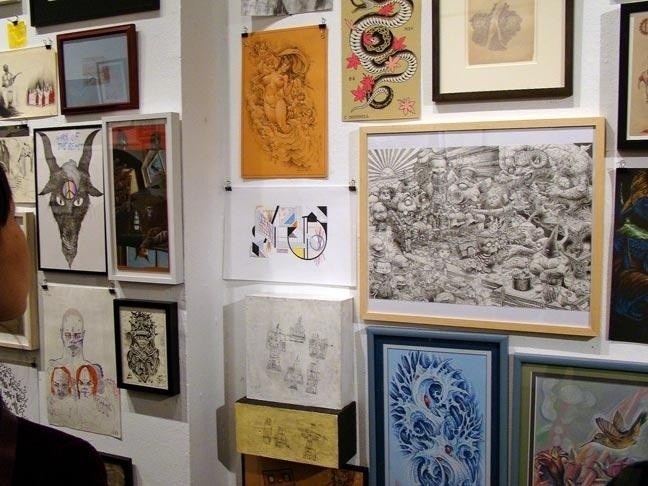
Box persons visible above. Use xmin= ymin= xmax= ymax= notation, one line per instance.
xmin=1 ymin=165 xmax=108 ymax=484
xmin=260 ymin=53 xmax=295 ymax=132
xmin=1 ymin=63 xmax=14 ymax=108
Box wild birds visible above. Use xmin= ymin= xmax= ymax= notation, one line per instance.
xmin=578 ymin=408 xmax=648 ymax=450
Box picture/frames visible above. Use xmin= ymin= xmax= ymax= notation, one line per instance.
xmin=0 ymin=211 xmax=38 ymax=350
xmin=616 ymin=1 xmax=648 ymax=150
xmin=55 ymin=23 xmax=136 ymax=114
xmin=96 ymin=450 xmax=133 ymax=486
xmin=358 ymin=116 xmax=607 ymax=337
xmin=369 ymin=326 xmax=508 ymax=486
xmin=102 ymin=113 xmax=183 ymax=285
xmin=241 ymin=453 xmax=368 ymax=486
xmin=113 ymin=298 xmax=179 ymax=395
xmin=510 ymin=351 xmax=647 ymax=486
xmin=433 ymin=0 xmax=574 ymax=104
xmin=32 ymin=121 xmax=107 ymax=275
